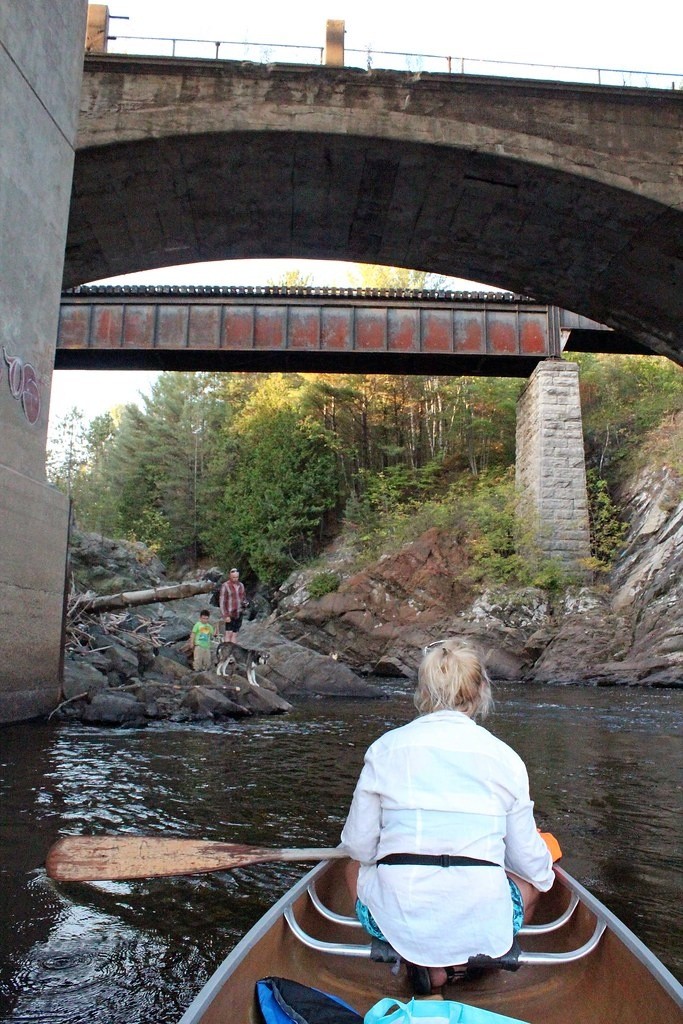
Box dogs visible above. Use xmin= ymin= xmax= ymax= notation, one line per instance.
xmin=214 ymin=642 xmax=271 ymax=688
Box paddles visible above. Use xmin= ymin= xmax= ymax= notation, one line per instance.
xmin=46 ymin=834 xmax=352 ymax=882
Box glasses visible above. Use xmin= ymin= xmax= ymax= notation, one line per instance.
xmin=231 ymin=570 xmax=239 ymax=573
xmin=426 ymin=640 xmax=470 ymax=659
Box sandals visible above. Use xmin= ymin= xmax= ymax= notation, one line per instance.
xmin=405 ymin=957 xmax=453 ymax=993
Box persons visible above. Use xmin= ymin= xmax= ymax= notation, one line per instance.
xmin=341 ymin=638 xmax=558 ymax=997
xmin=218 ymin=568 xmax=246 ymax=644
xmin=188 ymin=609 xmax=220 ymax=673
